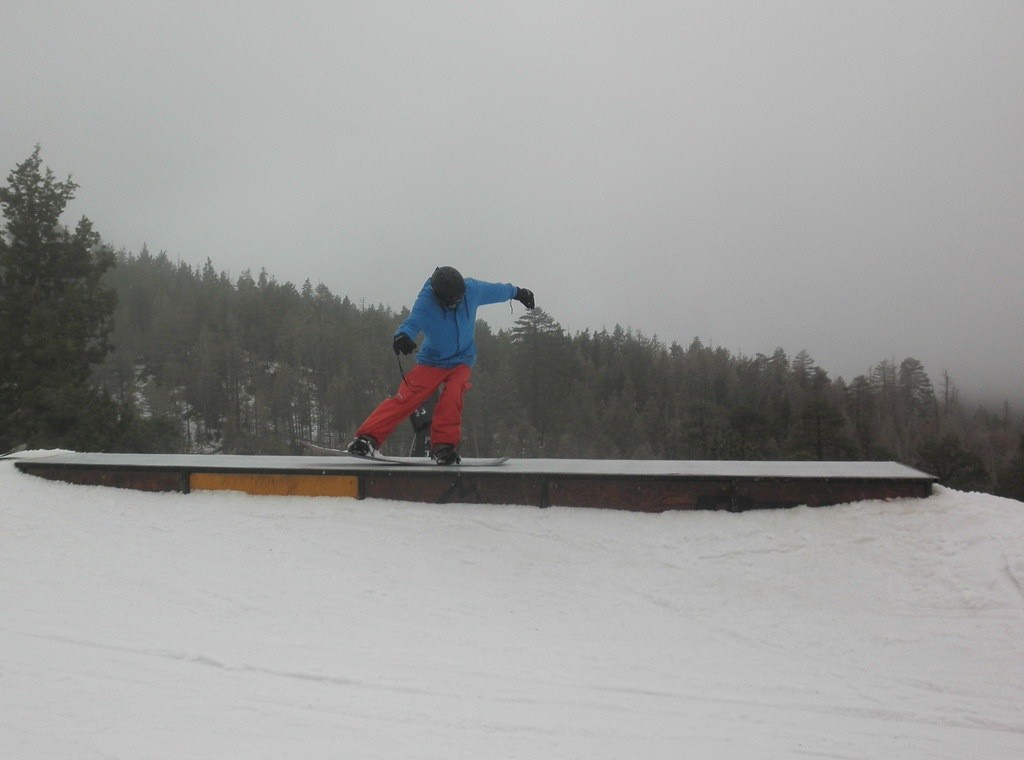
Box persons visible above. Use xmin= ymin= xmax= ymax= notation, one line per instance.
xmin=347 ymin=266 xmax=535 ymax=466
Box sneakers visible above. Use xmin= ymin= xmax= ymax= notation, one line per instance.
xmin=349 ymin=438 xmax=376 ymax=456
xmin=430 ymin=444 xmax=457 ymax=464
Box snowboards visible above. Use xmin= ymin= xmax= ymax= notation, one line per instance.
xmin=299 ymin=439 xmax=513 ymax=468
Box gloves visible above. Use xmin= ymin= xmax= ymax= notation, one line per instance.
xmin=393 ymin=334 xmax=416 ymax=355
xmin=513 ymin=287 xmax=535 ymax=310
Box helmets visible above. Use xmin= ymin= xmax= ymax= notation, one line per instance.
xmin=431 ymin=267 xmax=466 ymax=301
